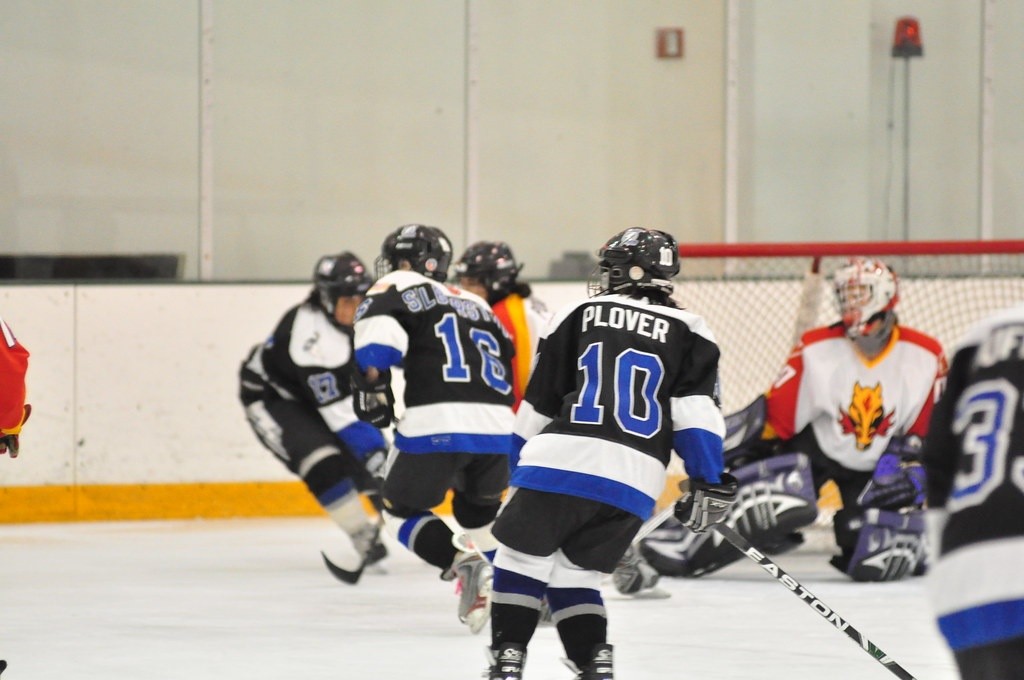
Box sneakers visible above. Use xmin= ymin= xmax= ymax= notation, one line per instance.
xmin=560 ymin=644 xmax=614 ymax=680
xmin=452 ymin=555 xmax=495 ymax=633
xmin=486 ymin=646 xmax=528 ymax=679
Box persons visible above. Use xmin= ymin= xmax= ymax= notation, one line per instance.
xmin=924 ymin=302 xmax=1024 ymax=680
xmin=480 ymin=226 xmax=735 ymax=680
xmin=239 ymin=252 xmax=391 ymax=565
xmin=0 ymin=317 xmax=32 ymax=680
xmin=723 ymin=261 xmax=947 ymax=578
xmin=354 ymin=225 xmax=557 ymax=625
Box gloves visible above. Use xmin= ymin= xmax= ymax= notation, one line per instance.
xmin=351 ymin=383 xmax=396 ymax=429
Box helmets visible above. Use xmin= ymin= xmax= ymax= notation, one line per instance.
xmin=832 ymin=262 xmax=899 ymax=335
xmin=597 ymin=228 xmax=680 ymax=295
xmin=313 ymin=252 xmax=377 ymax=316
xmin=454 ymin=241 xmax=524 ymax=299
xmin=382 ymin=223 xmax=452 ymax=285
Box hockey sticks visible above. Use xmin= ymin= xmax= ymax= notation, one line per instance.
xmin=319 ymin=517 xmax=385 ymax=588
xmin=349 ymin=359 xmax=401 ymax=427
xmin=678 ymin=491 xmax=917 ymax=680
